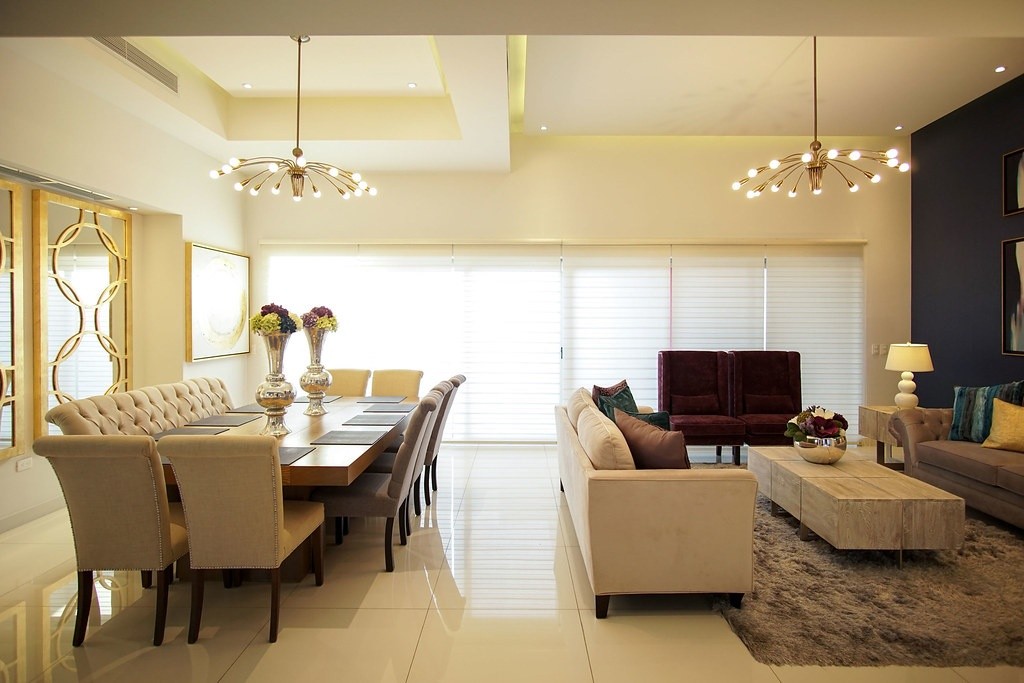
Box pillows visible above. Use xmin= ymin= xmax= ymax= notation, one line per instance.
xmin=603 ymin=403 xmax=670 ymax=431
xmin=979 ymin=397 xmax=1024 ymax=452
xmin=597 ymin=385 xmax=639 ymax=416
xmin=575 ymin=405 xmax=636 ymax=469
xmin=947 ymin=379 xmax=1024 ymax=441
xmin=592 ymin=379 xmax=628 ymax=402
xmin=613 ymin=407 xmax=690 ymax=469
xmin=566 ymin=386 xmax=598 ymax=431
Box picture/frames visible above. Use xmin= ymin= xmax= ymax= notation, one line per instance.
xmin=185 ymin=241 xmax=252 ymax=362
xmin=1000 ymin=236 xmax=1024 ymax=358
xmin=1000 ymin=145 xmax=1024 ymax=218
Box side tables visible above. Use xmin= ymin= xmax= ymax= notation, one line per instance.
xmin=857 ymin=405 xmax=925 ymax=471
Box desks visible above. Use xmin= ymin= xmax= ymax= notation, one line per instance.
xmin=151 ymin=395 xmax=427 ymax=587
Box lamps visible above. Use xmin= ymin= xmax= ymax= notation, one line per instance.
xmin=209 ymin=34 xmax=378 ymax=202
xmin=730 ymin=36 xmax=911 ymax=198
xmin=883 ymin=341 xmax=936 ymax=412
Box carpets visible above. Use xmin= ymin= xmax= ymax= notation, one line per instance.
xmin=711 ymin=489 xmax=1024 ymax=668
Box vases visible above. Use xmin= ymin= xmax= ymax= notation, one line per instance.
xmin=255 ymin=333 xmax=294 ymax=435
xmin=299 ymin=329 xmax=333 ymax=417
xmin=794 ymin=436 xmax=853 ymax=465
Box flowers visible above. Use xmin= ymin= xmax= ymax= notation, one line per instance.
xmin=300 ymin=305 xmax=339 ymax=331
xmin=783 ymin=403 xmax=848 ymax=441
xmin=249 ymin=302 xmax=301 ymax=336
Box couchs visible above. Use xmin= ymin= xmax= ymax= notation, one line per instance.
xmin=552 ymin=403 xmax=758 ymax=619
xmin=886 ymin=408 xmax=1024 ymax=531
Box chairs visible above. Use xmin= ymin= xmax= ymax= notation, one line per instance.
xmin=656 ymin=348 xmax=743 ymax=465
xmin=730 ymin=349 xmax=805 ymax=447
xmin=32 ymin=363 xmax=470 ymax=651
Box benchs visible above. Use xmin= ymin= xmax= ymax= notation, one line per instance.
xmin=45 ymin=375 xmax=236 ymax=588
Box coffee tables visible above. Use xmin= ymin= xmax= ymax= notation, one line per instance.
xmin=747 ymin=445 xmax=968 ymax=567
